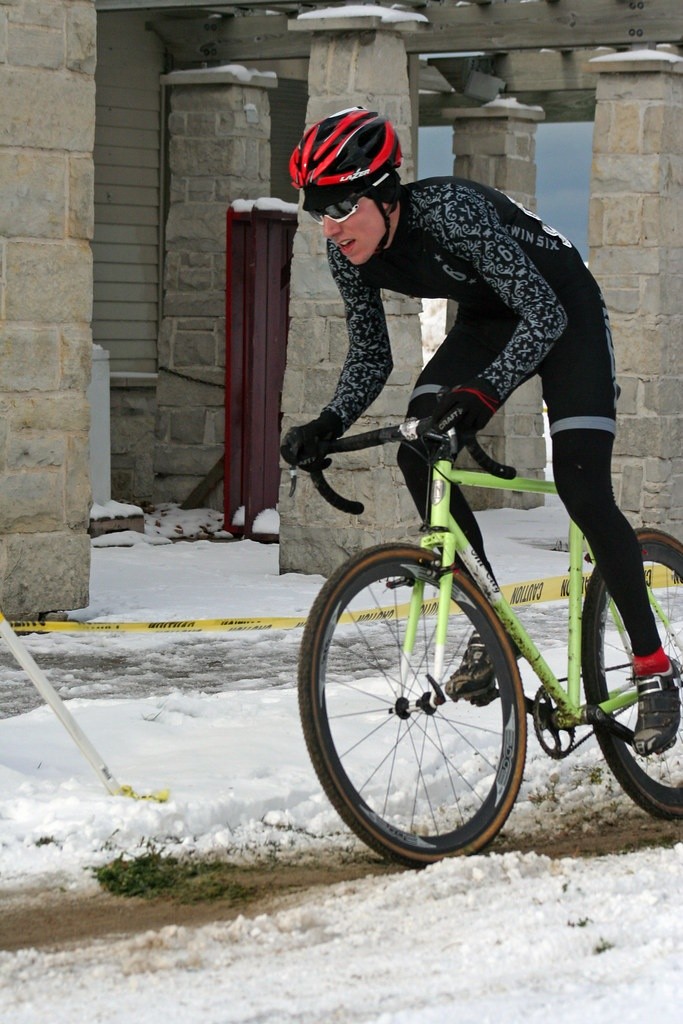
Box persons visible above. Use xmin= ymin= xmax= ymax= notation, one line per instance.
xmin=281 ymin=107 xmax=683 ymax=756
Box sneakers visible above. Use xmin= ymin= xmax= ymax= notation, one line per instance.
xmin=632 ymin=656 xmax=683 ymax=757
xmin=444 ymin=620 xmax=521 ymax=701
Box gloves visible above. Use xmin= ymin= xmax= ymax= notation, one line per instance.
xmin=431 ymin=378 xmax=501 ymax=433
xmin=279 ymin=410 xmax=345 ymax=472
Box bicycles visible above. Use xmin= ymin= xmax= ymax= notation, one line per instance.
xmin=287 ymin=386 xmax=683 ymax=870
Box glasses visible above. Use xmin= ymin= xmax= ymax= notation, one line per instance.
xmin=307 ymin=189 xmax=373 ymax=226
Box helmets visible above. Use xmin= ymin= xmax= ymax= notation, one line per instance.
xmin=288 ymin=106 xmax=403 ymax=212
